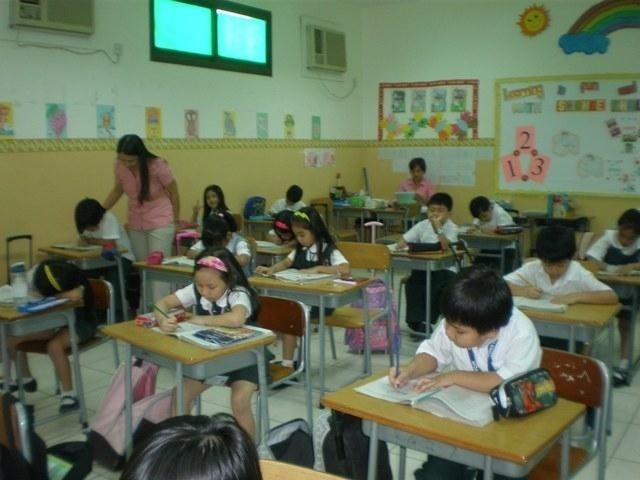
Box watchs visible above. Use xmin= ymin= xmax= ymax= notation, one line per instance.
xmin=433 ymin=227 xmax=445 ymax=238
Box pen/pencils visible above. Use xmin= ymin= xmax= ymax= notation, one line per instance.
xmin=517 ymin=274 xmax=534 ymax=287
xmin=393 ymin=335 xmax=399 ymax=389
xmin=153 ymin=304 xmax=182 ymax=329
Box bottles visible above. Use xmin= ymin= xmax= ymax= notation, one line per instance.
xmin=335 ymin=173 xmax=345 ymax=201
xmin=10 ymin=261 xmax=29 ymax=307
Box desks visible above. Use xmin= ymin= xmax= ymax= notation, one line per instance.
xmin=255 ymin=243 xmax=293 ymax=266
xmin=132 ymin=256 xmax=196 ymax=314
xmin=317 ymin=366 xmax=586 ymax=480
xmin=247 ymin=272 xmax=376 ymax=411
xmin=169 ymin=216 xmax=279 ymax=250
xmin=38 ymin=242 xmax=129 ymax=321
xmin=97 ymin=311 xmax=275 ymax=450
xmin=333 ymin=202 xmax=410 ymax=239
xmin=453 ymin=225 xmax=523 ymax=276
xmin=392 ymin=248 xmax=467 ymax=342
xmin=0 ymin=286 xmax=89 ymax=440
xmin=575 ymin=260 xmax=639 ymax=379
xmin=521 ymin=209 xmax=598 ymax=259
xmin=519 ymin=300 xmax=623 ymax=434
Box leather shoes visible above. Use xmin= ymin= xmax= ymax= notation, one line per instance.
xmin=1 ymin=378 xmax=39 ymax=393
xmin=60 ymin=395 xmax=80 ymax=412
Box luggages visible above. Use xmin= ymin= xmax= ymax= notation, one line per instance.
xmin=346 ymin=221 xmax=400 ymax=355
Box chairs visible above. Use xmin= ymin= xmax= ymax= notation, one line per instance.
xmin=309 ymin=198 xmax=359 ymax=242
xmin=296 ymin=239 xmax=394 ymax=376
xmin=14 ymin=280 xmax=118 ymax=417
xmin=228 ymin=212 xmax=243 ymax=236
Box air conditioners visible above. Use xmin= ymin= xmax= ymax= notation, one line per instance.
xmin=9 ymin=0 xmax=96 ymax=37
xmin=306 ymin=23 xmax=348 ymax=73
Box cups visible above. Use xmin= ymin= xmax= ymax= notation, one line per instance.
xmin=351 ymin=197 xmax=364 ymax=207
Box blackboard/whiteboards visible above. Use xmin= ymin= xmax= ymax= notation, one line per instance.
xmin=493 ymin=73 xmax=640 ymax=199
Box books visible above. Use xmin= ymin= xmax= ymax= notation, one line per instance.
xmin=510 ymin=291 xmax=568 ymax=315
xmin=254 ymin=269 xmax=333 ymax=286
xmin=353 ymin=368 xmax=494 ymax=427
xmin=152 ymin=318 xmax=274 ymax=352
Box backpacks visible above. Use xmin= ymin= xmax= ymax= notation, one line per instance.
xmin=0 ymin=393 xmax=92 ymax=480
xmin=266 ymin=408 xmax=392 ymax=480
xmin=87 ymin=356 xmax=176 ymax=471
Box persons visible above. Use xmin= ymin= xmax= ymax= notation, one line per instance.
xmin=183 ymin=212 xmax=252 ymax=277
xmin=252 ymin=206 xmax=352 ymax=370
xmin=581 ymin=206 xmax=640 ymax=382
xmin=194 ymin=185 xmax=233 ymax=232
xmin=398 ymin=193 xmax=459 ymax=337
xmin=264 ymin=210 xmax=300 ymax=249
xmin=267 ymin=185 xmax=307 ymax=212
xmin=152 ymin=247 xmax=276 ymax=444
xmin=76 ymin=199 xmax=134 ymax=312
xmin=465 ymin=194 xmax=516 ymax=274
xmin=386 ymin=263 xmax=544 ymax=478
xmin=0 ymin=260 xmax=98 ymax=413
xmin=498 ymin=224 xmax=619 ymax=305
xmin=121 ymin=411 xmax=262 ymax=479
xmin=397 ymin=158 xmax=435 ymax=209
xmin=101 ymin=133 xmax=180 ymax=314
